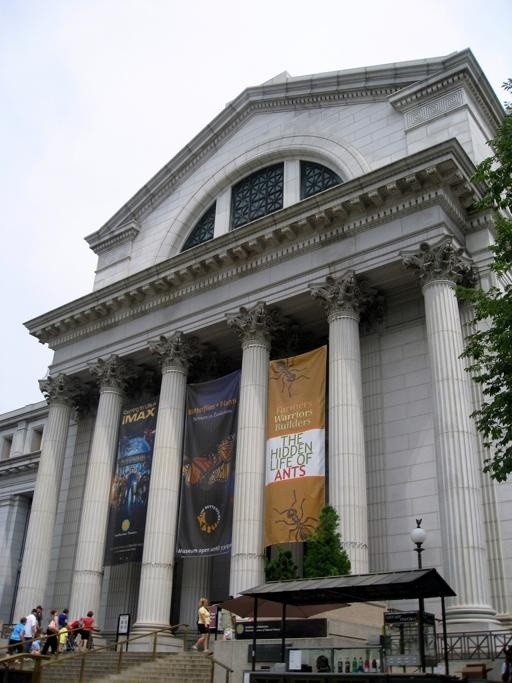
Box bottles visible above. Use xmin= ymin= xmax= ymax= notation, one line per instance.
xmin=338 ymin=656 xmax=378 ymax=673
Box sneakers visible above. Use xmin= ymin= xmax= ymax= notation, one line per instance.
xmin=203 ymin=650 xmax=213 ymax=655
xmin=191 ymin=645 xmax=197 ymax=652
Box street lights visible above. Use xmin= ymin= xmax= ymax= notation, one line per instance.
xmin=408 ymin=515 xmax=429 ymax=674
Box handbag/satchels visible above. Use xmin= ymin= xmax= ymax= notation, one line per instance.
xmin=48 ymin=620 xmax=59 ymax=633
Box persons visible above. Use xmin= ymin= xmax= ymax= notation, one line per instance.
xmin=6 ymin=605 xmax=97 ymax=665
xmin=192 ymin=597 xmax=214 ymax=656
xmin=316 ymin=655 xmax=332 ymax=673
xmin=110 ymin=467 xmax=142 ymax=506
xmin=501 ymin=646 xmax=511 ymax=683
